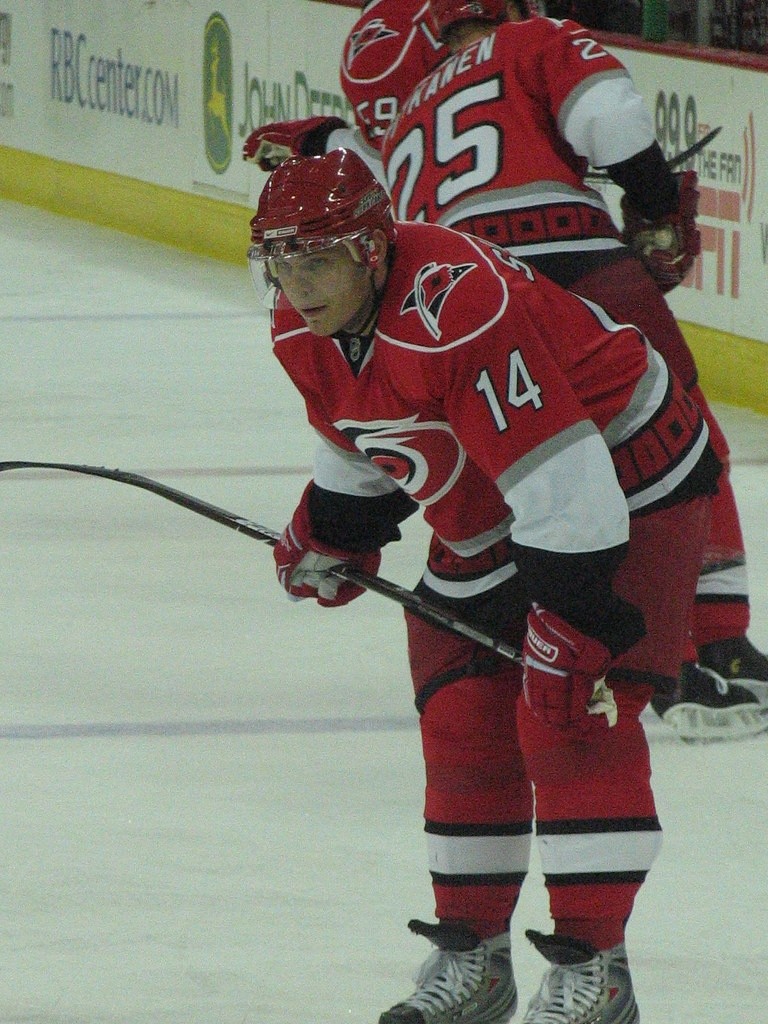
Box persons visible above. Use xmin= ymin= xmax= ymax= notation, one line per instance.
xmin=246 ymin=151 xmax=724 ymax=1022
xmin=337 ymin=1 xmax=768 ymax=747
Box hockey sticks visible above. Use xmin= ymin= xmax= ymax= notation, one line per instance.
xmin=651 ymin=228 xmax=674 ymax=250
xmin=0 ymin=460 xmax=525 ymax=664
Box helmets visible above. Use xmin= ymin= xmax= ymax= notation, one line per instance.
xmin=429 ymin=0 xmax=540 ymax=42
xmin=246 ymin=147 xmax=398 ymax=309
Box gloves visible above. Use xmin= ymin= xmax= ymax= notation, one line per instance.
xmin=621 ymin=171 xmax=702 ymax=296
xmin=273 ymin=479 xmax=419 ymax=608
xmin=243 ymin=117 xmax=347 ymax=172
xmin=516 ymin=596 xmax=648 ymax=787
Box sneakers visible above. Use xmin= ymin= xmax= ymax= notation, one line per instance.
xmin=696 ymin=638 xmax=768 ymax=688
xmin=378 ymin=919 xmax=518 ymax=1024
xmin=651 ymin=661 xmax=768 ymax=744
xmin=523 ymin=929 xmax=641 ymax=1024
